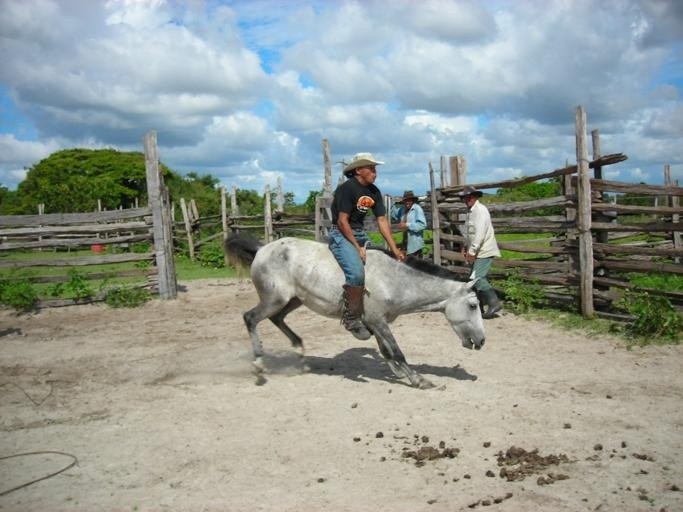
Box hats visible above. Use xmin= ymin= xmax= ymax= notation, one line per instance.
xmin=456 ymin=186 xmax=483 ymax=200
xmin=399 ymin=189 xmax=419 ymax=205
xmin=343 ymin=151 xmax=384 ymax=177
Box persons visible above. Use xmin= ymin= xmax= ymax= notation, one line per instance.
xmin=397 ymin=191 xmax=426 ymax=259
xmin=391 ymin=197 xmax=404 ymax=223
xmin=462 ymin=185 xmax=503 ymax=319
xmin=327 ymin=152 xmax=405 ymax=341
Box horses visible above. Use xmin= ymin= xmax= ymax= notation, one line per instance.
xmin=224 ymin=233 xmax=486 ymax=390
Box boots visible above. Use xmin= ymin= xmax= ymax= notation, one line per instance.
xmin=479 ymin=288 xmax=503 ymax=319
xmin=340 ymin=285 xmax=372 ymax=341
